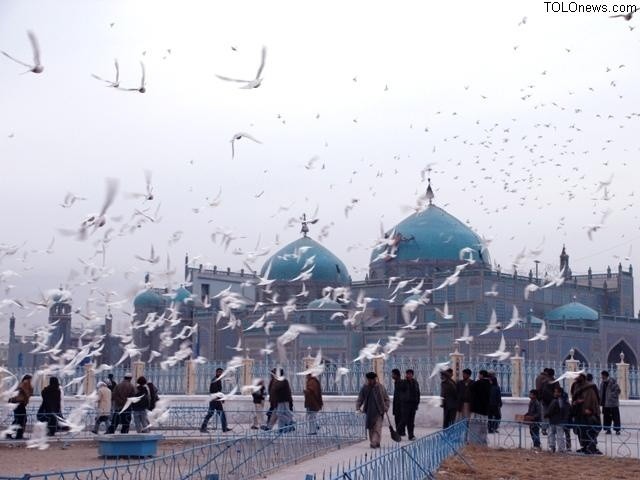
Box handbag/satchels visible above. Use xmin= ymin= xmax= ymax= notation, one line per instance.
xmin=37 ymin=388 xmax=48 ymax=422
xmin=385 ymin=408 xmax=402 ymax=445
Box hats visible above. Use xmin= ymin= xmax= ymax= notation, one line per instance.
xmin=366 ymin=372 xmax=377 ymax=379
xmin=441 ymin=371 xmax=452 ymax=376
xmin=124 ymin=373 xmax=132 ymax=377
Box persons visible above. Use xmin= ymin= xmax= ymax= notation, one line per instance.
xmin=356 ymin=372 xmax=391 ymax=447
xmin=37 ymin=377 xmax=70 ymax=436
xmin=6 ymin=375 xmax=33 ymax=439
xmin=536 ymin=369 xmax=549 ymax=391
xmin=268 ymin=370 xmax=297 ymax=433
xmin=132 ymin=376 xmax=151 ymax=433
xmin=441 ymin=371 xmax=455 ymax=428
xmin=599 ymin=372 xmax=622 ymax=435
xmin=543 ymin=368 xmax=557 ymax=393
xmin=572 ymin=374 xmax=601 ymax=454
xmin=105 ymin=374 xmax=117 ymax=389
xmin=560 ymin=391 xmax=571 ymax=451
xmin=92 ymin=382 xmax=112 ymax=436
xmin=405 ymin=369 xmax=419 ymax=442
xmin=103 ymin=374 xmax=135 ymax=435
xmin=391 ymin=369 xmax=407 ymax=436
xmin=525 ymin=389 xmax=541 ymax=449
xmin=487 ymin=368 xmax=502 ymax=431
xmin=250 ymin=380 xmax=268 ymax=430
xmin=201 ymin=367 xmax=232 ymax=433
xmin=304 ymin=373 xmax=324 ymax=436
xmin=473 ymin=371 xmax=488 ymax=435
xmin=457 ymin=369 xmax=469 ymax=441
xmin=545 ymin=387 xmax=565 ymax=451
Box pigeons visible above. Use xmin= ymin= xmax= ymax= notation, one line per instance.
xmin=209 ymin=383 xmax=238 ymax=402
xmin=241 ymin=378 xmax=262 ymax=393
xmin=90 ymin=58 xmax=125 ymax=93
xmin=479 ymin=309 xmax=503 ymax=336
xmin=524 ymin=319 xmax=550 ymax=344
xmin=482 ymin=282 xmax=501 ymax=298
xmin=422 ymin=320 xmax=440 ymax=338
xmin=282 ymin=66 xmax=479 ymax=321
xmin=211 ymin=174 xmax=283 ymax=381
xmin=501 ymin=304 xmax=524 ymax=331
xmin=0 ymin=174 xmax=211 ymax=450
xmin=541 ymin=261 xmax=568 ymax=290
xmin=279 ymin=323 xmax=317 ymax=346
xmin=199 ymin=293 xmax=210 ymax=312
xmin=477 ymin=4 xmax=640 ymax=261
xmin=548 ymin=366 xmax=585 ymax=385
xmin=334 ymin=321 xmax=417 ymax=380
xmin=427 ymin=360 xmax=452 ymax=378
xmin=1 ymin=25 xmax=46 ymax=77
xmin=477 ymin=333 xmax=511 ymax=363
xmin=523 ymin=280 xmax=541 ymax=300
xmin=453 ymin=322 xmax=475 ymax=346
xmin=295 ymin=348 xmax=327 ymax=380
xmin=228 ymin=131 xmax=264 ymax=161
xmin=118 ymin=57 xmax=150 ymax=95
xmin=216 ymin=47 xmax=267 ymax=90
